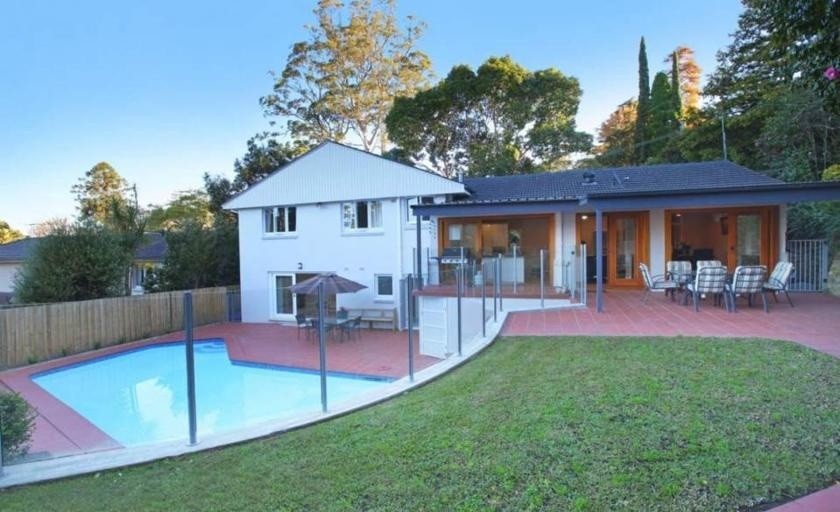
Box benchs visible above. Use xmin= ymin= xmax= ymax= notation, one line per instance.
xmin=346 ymin=308 xmax=396 ymax=332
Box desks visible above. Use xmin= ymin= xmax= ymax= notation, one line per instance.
xmin=480 ymin=255 xmax=525 ymax=285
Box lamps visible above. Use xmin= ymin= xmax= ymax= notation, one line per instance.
xmin=576 ymin=171 xmax=598 ymax=206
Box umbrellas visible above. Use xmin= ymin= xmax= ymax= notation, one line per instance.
xmin=290 ymin=272 xmax=370 ymax=321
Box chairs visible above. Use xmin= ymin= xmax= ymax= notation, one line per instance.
xmin=639 ymin=259 xmax=793 ymax=312
xmin=296 ymin=311 xmax=361 ymax=344
